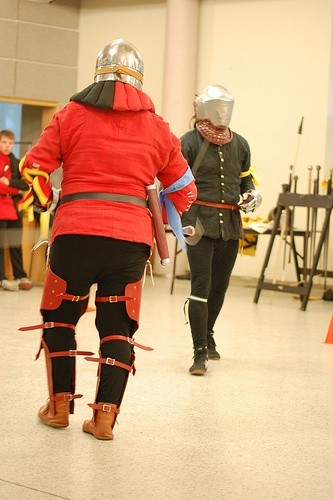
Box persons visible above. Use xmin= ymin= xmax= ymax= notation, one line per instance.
xmin=0 ymin=130 xmax=33 ymax=291
xmin=179 ymin=85 xmax=262 ymax=374
xmin=18 ymin=38 xmax=197 ymax=440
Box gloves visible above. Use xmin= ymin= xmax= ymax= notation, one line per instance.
xmin=239 ymin=190 xmax=262 ymax=213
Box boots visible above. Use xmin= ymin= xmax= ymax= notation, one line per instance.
xmin=83 ymin=402 xmax=117 ymax=440
xmin=39 ymin=392 xmax=71 ymax=427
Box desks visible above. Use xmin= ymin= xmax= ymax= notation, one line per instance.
xmin=164 ymin=229 xmax=311 ymax=295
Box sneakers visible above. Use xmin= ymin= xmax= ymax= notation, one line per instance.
xmin=207 ymin=337 xmax=220 ymax=360
xmin=189 ymin=350 xmax=207 ymax=374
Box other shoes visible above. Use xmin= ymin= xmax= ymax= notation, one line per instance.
xmin=18 ymin=278 xmax=33 ymax=290
xmin=0 ymin=280 xmax=18 ymax=291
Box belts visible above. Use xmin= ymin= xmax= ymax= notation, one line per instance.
xmin=59 ymin=193 xmax=148 ymax=207
xmin=193 ymin=200 xmax=238 ymax=210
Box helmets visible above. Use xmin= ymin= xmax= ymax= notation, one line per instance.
xmin=94 ymin=39 xmax=144 ymax=88
xmin=193 ymin=84 xmax=234 ymax=129
